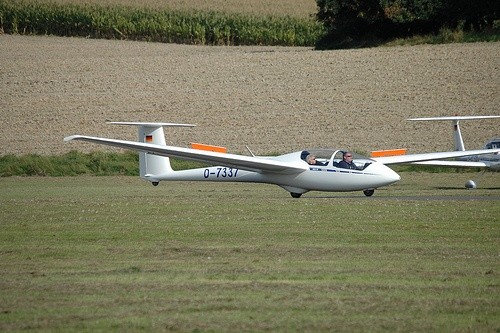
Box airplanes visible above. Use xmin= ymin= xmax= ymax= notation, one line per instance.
xmin=370 ymin=116 xmax=500 ymax=174
xmin=63 ymin=121 xmax=401 ymax=198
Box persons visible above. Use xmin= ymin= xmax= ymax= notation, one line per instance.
xmin=338 ymin=152 xmax=359 ymax=170
xmin=305 ymin=154 xmax=320 ymax=166
